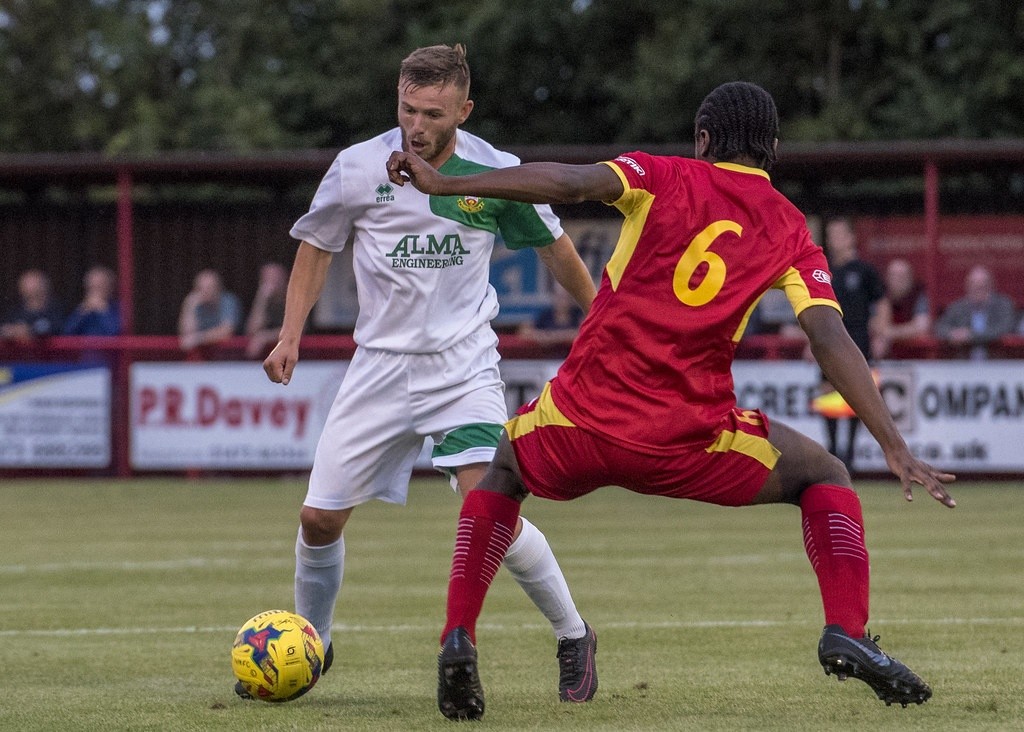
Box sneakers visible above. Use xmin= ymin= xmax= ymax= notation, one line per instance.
xmin=438 ymin=629 xmax=485 ymax=723
xmin=235 ymin=641 xmax=333 ymax=699
xmin=819 ymin=625 xmax=932 ymax=709
xmin=556 ymin=619 xmax=599 ymax=704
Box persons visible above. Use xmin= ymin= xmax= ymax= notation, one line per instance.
xmin=520 ymin=215 xmax=1023 ymax=469
xmin=177 ymin=263 xmax=309 ymax=358
xmin=386 ymin=81 xmax=957 ymax=721
xmin=0 ymin=265 xmax=121 ymax=338
xmin=234 ymin=42 xmax=599 ymax=703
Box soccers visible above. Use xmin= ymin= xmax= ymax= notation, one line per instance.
xmin=230 ymin=608 xmax=328 ymax=705
